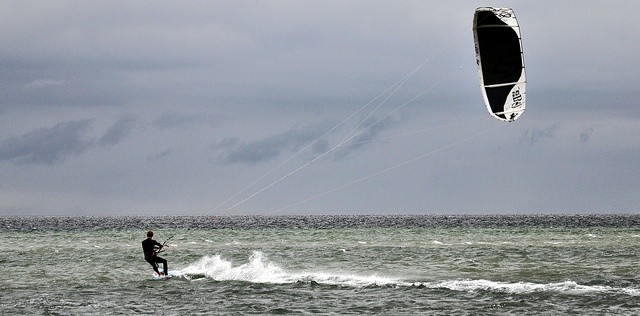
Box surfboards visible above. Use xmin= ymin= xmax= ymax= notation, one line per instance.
xmin=153 ymin=268 xmax=206 ymax=279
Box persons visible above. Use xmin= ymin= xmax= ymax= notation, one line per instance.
xmin=142 ymin=231 xmax=168 ymax=276
